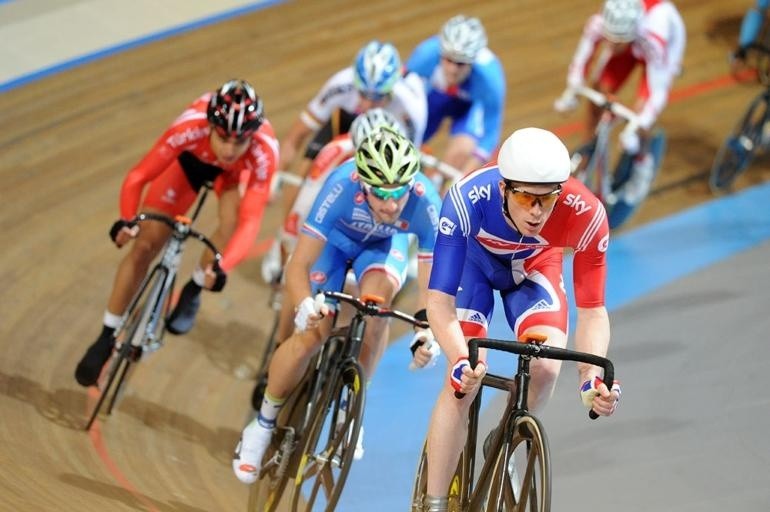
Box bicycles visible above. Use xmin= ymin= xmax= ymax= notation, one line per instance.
xmin=237 ymin=260 xmax=438 ymax=511
xmin=84 ymin=177 xmax=228 ymax=433
xmin=411 ymin=332 xmax=614 ymax=512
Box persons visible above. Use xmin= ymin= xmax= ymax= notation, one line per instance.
xmin=403 ymin=15 xmax=507 ymax=187
xmin=233 ymin=124 xmax=442 ymax=486
xmin=566 ymin=0 xmax=689 ymax=206
xmin=737 ymin=1 xmax=769 ymax=61
xmin=261 ymin=41 xmax=428 ymax=285
xmin=420 ymin=127 xmax=620 ymax=512
xmin=75 ymin=79 xmax=278 ymax=386
xmin=251 ymin=107 xmax=412 ymax=414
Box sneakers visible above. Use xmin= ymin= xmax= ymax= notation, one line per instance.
xmin=166 ymin=280 xmax=203 ymax=336
xmin=618 ymin=152 xmax=658 ymax=210
xmin=74 ymin=331 xmax=119 ymax=387
xmin=231 ymin=418 xmax=275 ymax=485
xmin=261 ymin=236 xmax=284 ymax=285
xmin=347 ymin=425 xmax=365 ymax=462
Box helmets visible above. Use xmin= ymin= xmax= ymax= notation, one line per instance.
xmin=440 ymin=14 xmax=488 ymax=64
xmin=354 ymin=39 xmax=402 ymax=94
xmin=496 ymin=126 xmax=572 ymax=184
xmin=355 ymin=127 xmax=421 ymax=185
xmin=349 ymin=107 xmax=409 ymax=150
xmin=597 ymin=0 xmax=649 ymax=45
xmin=205 ymin=79 xmax=264 ymax=140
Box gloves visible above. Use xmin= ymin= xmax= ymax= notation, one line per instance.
xmin=449 ymin=357 xmax=487 ymax=395
xmin=108 ymin=218 xmax=142 ymax=248
xmin=210 ymin=263 xmax=229 ymax=294
xmin=408 ymin=331 xmax=444 ymax=370
xmin=293 ymin=295 xmax=329 ymax=333
xmin=580 ymin=374 xmax=622 ymax=420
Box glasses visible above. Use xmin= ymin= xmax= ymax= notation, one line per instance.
xmin=362 ymin=182 xmax=415 ymax=201
xmin=504 ymin=183 xmax=564 ymax=210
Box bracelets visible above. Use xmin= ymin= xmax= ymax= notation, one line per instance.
xmin=415 ymin=310 xmax=426 ymax=322
